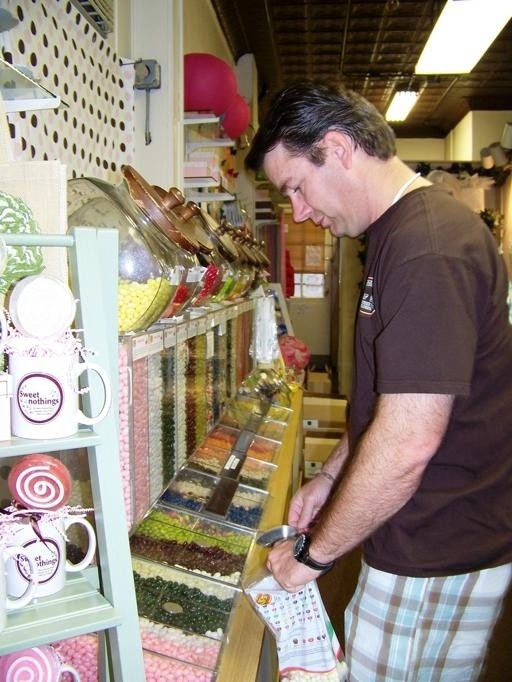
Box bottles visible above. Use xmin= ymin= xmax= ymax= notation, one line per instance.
xmin=67 ymin=163 xmax=270 ymax=342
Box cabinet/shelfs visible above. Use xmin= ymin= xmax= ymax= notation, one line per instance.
xmin=444 ymin=110 xmax=512 ymax=163
xmin=0 ymin=225 xmax=147 ymax=682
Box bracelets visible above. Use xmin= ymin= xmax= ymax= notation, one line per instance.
xmin=322 ymin=471 xmax=337 ymax=484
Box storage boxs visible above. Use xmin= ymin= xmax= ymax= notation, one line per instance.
xmin=306 ymin=361 xmax=334 ymax=396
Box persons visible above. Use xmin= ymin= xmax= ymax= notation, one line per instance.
xmin=244 ymin=83 xmax=512 ymax=682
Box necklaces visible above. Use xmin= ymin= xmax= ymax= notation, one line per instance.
xmin=391 ymin=172 xmax=423 ymax=206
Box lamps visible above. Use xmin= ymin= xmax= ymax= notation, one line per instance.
xmin=385 ymin=90 xmax=418 ymax=122
xmin=414 ymin=0 xmax=512 ymax=75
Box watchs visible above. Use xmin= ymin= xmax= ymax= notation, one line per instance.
xmin=292 ymin=534 xmax=333 ymax=571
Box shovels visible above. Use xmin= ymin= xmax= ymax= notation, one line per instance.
xmin=256 ymin=525 xmax=316 ymax=548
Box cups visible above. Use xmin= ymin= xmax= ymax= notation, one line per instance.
xmin=53 ymin=650 xmax=85 ymax=682
xmin=0 ymin=542 xmax=40 ymax=634
xmin=8 ymin=350 xmax=114 ymax=442
xmin=480 ymin=121 xmax=511 ymax=169
xmin=7 ymin=509 xmax=97 ymax=599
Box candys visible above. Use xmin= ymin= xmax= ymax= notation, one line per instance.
xmin=0 ymin=267 xmax=279 ymax=682
